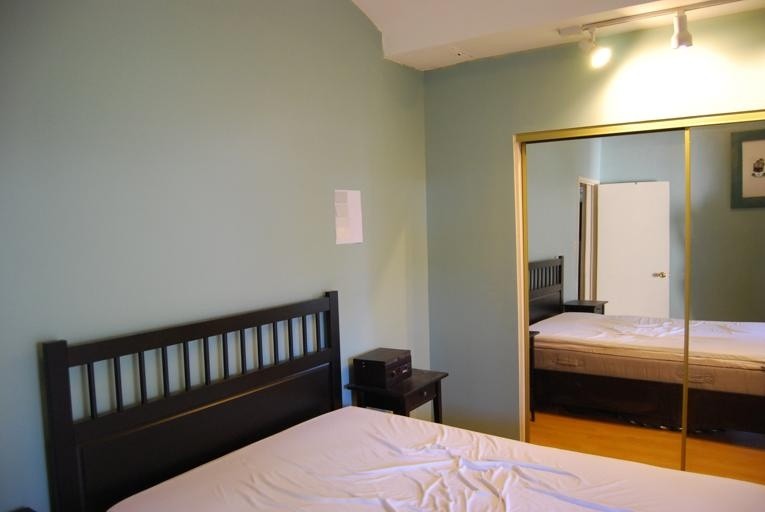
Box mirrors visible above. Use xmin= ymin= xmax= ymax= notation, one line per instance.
xmin=516 ymin=109 xmax=765 ymax=486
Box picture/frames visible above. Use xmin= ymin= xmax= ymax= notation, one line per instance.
xmin=730 ymin=128 xmax=765 ymax=209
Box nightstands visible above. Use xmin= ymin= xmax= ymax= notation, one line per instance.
xmin=564 ymin=300 xmax=608 ymax=315
xmin=343 ymin=367 xmax=449 ymax=427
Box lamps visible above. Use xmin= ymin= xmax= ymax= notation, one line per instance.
xmin=671 ymin=9 xmax=694 ymax=50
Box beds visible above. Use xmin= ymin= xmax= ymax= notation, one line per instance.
xmin=35 ymin=289 xmax=765 ymax=512
xmin=527 ymin=255 xmax=765 ymax=436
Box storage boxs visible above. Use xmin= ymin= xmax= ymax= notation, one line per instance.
xmin=353 ymin=347 xmax=412 ymax=388
